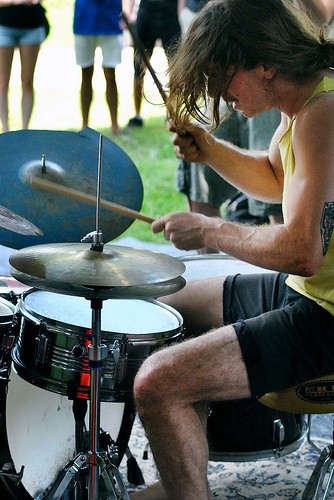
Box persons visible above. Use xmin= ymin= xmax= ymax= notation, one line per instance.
xmin=74 ymin=0 xmax=334 ymax=254
xmin=0 ymin=1 xmax=51 ymax=132
xmin=133 ymin=0 xmax=334 ymax=500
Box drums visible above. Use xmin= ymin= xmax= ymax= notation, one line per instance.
xmin=10 ymin=286 xmax=186 ymax=403
xmin=0 ymin=296 xmax=17 ymax=383
xmin=0 ymin=361 xmax=137 ymax=500
xmin=203 ymin=396 xmax=310 ymax=463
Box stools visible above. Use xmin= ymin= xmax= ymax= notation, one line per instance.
xmin=256 ymin=373 xmax=334 ymax=500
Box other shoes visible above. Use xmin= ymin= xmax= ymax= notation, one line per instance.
xmin=127 ymin=118 xmax=142 ymax=127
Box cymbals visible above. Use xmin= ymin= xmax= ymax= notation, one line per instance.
xmin=9 ymin=242 xmax=187 ymax=300
xmin=0 ymin=126 xmax=144 ymax=250
